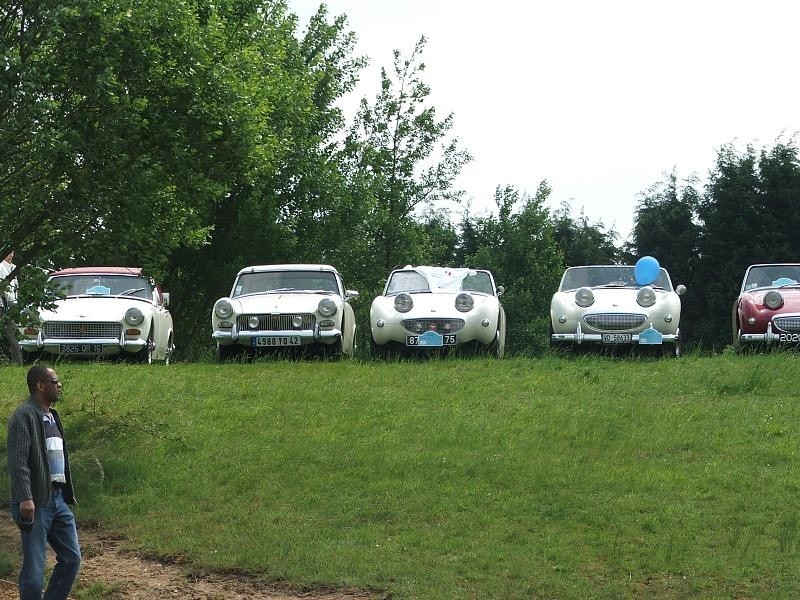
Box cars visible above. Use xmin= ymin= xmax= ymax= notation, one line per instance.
xmin=549 ymin=264 xmax=687 ymax=360
xmin=730 ymin=263 xmax=800 ymax=350
xmin=211 ymin=264 xmax=359 ymax=361
xmin=17 ymin=265 xmax=176 ymax=367
xmin=369 ymin=266 xmax=507 ymax=357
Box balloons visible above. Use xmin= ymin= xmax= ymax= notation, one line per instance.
xmin=634 ymin=256 xmax=661 ymax=286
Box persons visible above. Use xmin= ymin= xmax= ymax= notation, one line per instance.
xmin=0 ymin=246 xmax=18 ymax=314
xmin=7 ymin=366 xmax=82 ymax=600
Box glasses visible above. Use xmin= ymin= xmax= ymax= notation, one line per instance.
xmin=40 ymin=378 xmax=59 ymax=384
